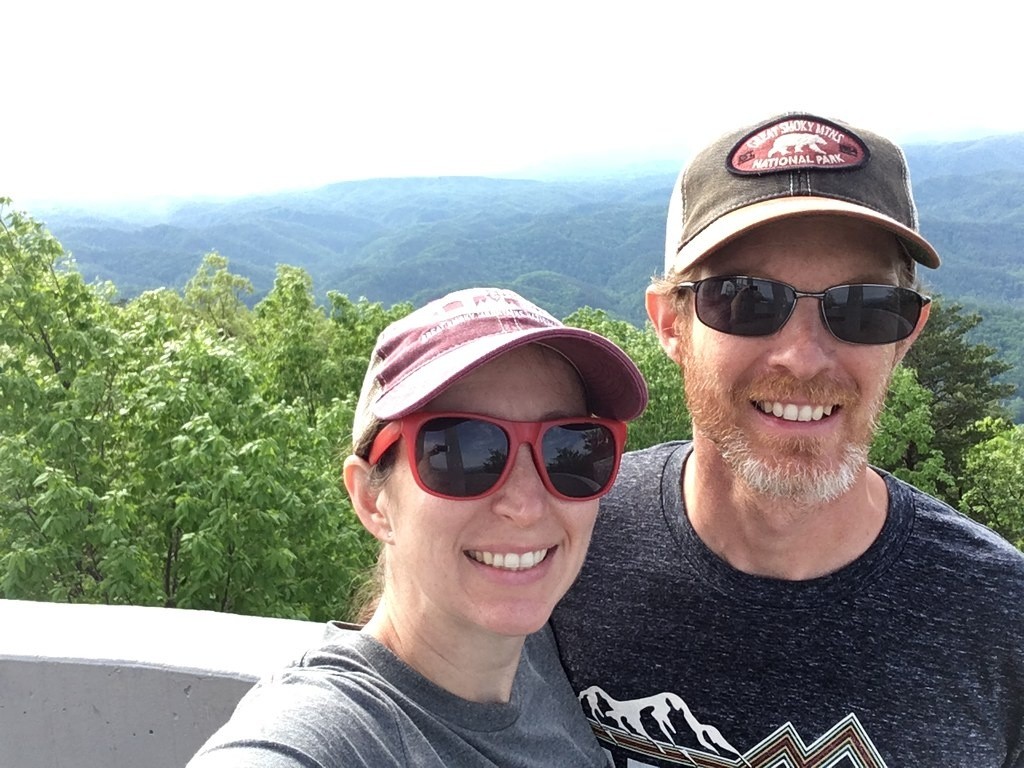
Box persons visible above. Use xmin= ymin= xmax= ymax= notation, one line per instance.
xmin=184 ymin=286 xmax=648 ymax=768
xmin=550 ymin=111 xmax=1024 ymax=768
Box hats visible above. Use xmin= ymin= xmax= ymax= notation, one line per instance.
xmin=664 ymin=112 xmax=941 ymax=276
xmin=351 ymin=289 xmax=650 ymax=465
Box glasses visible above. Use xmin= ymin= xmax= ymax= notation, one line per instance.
xmin=674 ymin=273 xmax=932 ymax=346
xmin=368 ymin=412 xmax=627 ymax=503
